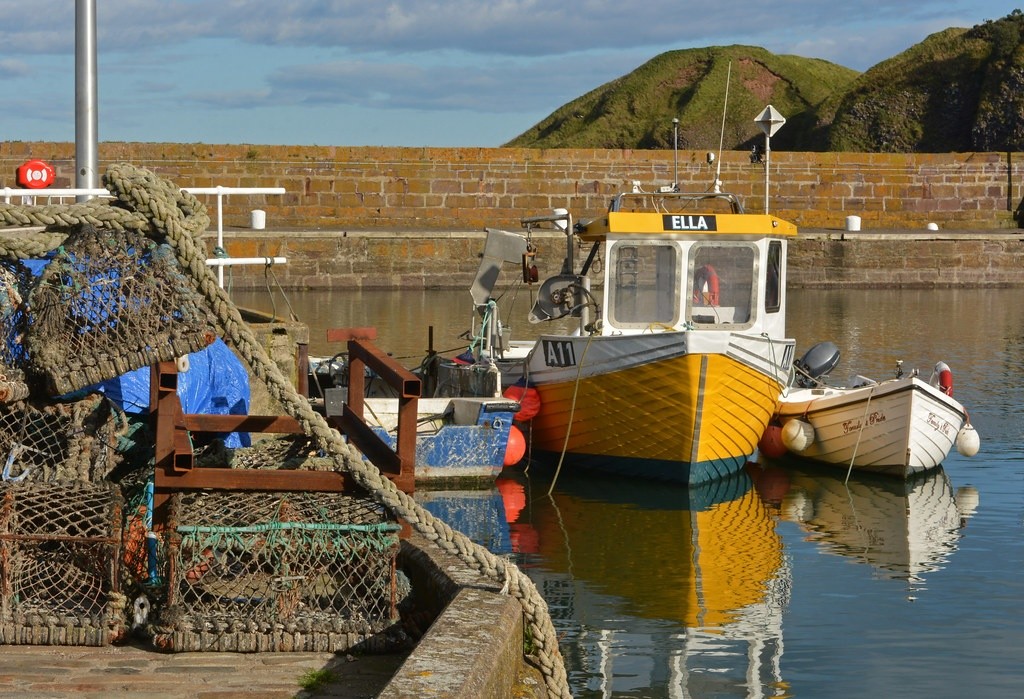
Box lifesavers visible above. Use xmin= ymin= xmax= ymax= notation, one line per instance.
xmin=693 ymin=264 xmax=720 ymax=305
xmin=935 ymin=360 xmax=953 ymax=397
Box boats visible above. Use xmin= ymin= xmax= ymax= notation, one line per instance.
xmin=305 ymin=325 xmax=523 ymax=488
xmin=756 ymin=341 xmax=981 ymax=482
xmin=457 ymin=61 xmax=801 ymax=487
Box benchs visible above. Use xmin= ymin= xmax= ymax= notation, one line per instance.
xmin=364 ymin=397 xmax=453 ymax=414
